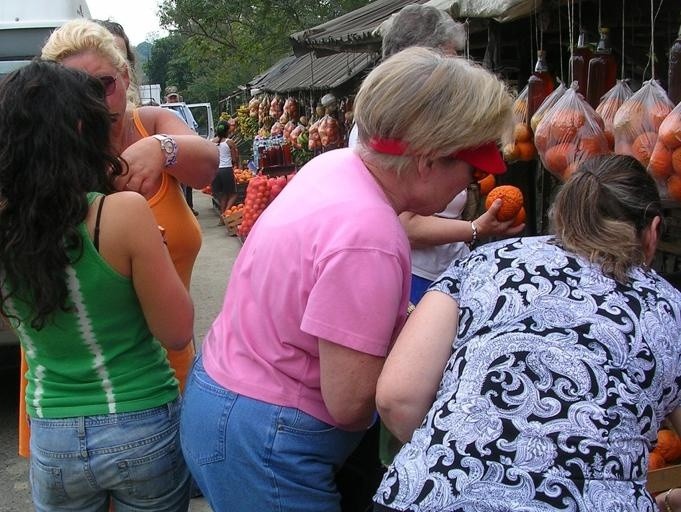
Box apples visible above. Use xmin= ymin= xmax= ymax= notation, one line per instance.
xmin=248 ymin=96 xmax=339 ymax=152
xmin=238 ymin=178 xmax=287 ymax=236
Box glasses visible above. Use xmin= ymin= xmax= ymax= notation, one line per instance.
xmin=99 ymin=75 xmax=118 ymax=96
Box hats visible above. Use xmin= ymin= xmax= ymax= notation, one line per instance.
xmin=320 ymin=92 xmax=338 ymax=110
xmin=369 ymin=129 xmax=507 ymax=174
xmin=164 ymin=86 xmax=178 ymax=97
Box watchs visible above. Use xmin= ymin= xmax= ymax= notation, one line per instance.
xmin=152 ymin=134 xmax=178 ymax=168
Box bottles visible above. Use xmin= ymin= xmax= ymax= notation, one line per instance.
xmin=252 ymin=134 xmax=292 ymax=171
xmin=527 ymin=46 xmax=554 ymax=140
xmin=567 ymin=27 xmax=618 ymax=108
xmin=667 ymin=25 xmax=681 ymax=106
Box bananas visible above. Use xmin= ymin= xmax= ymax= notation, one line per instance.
xmin=220 ymin=105 xmax=258 ymax=137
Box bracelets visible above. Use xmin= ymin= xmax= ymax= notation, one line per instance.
xmin=665 ymin=488 xmax=673 ymax=512
xmin=465 ymin=221 xmax=478 ymax=247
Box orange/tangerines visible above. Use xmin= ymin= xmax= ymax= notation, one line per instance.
xmin=233 ymin=168 xmax=252 ymax=183
xmin=649 ymin=429 xmax=680 ymax=471
xmin=475 ymin=96 xmax=681 ymax=226
xmin=224 ymin=204 xmax=243 ymax=223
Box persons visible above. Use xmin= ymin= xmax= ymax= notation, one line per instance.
xmin=177 ymin=45 xmax=519 ymax=512
xmin=0 ymin=59 xmax=195 ymax=512
xmin=90 ymin=19 xmax=135 ymax=66
xmin=347 ymin=2 xmax=527 ymax=306
xmin=164 ymin=85 xmax=200 ymax=217
xmin=210 ymin=120 xmax=239 ymax=227
xmin=17 ymin=18 xmax=222 ymax=458
xmin=363 ymin=151 xmax=681 ymax=512
